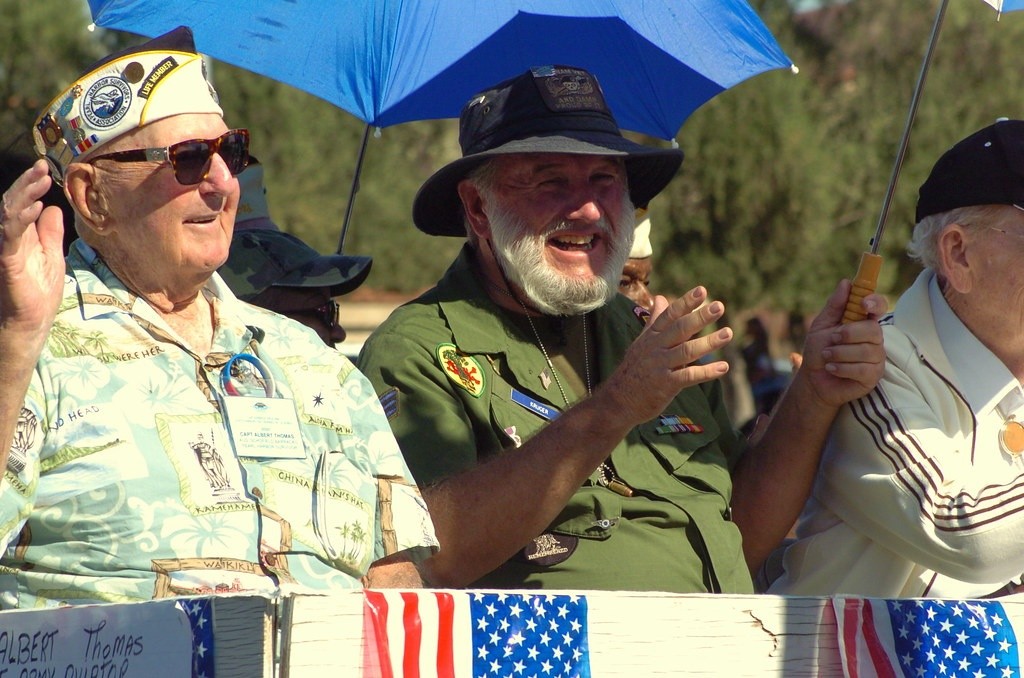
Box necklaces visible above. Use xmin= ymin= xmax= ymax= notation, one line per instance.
xmin=519 ymin=295 xmax=636 ymax=499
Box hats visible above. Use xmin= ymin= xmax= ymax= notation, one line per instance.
xmin=626 ymin=207 xmax=653 ymax=259
xmin=233 ymin=163 xmax=281 ymax=232
xmin=411 ymin=68 xmax=685 ymax=238
xmin=33 ymin=25 xmax=224 ymax=184
xmin=216 ymin=231 xmax=373 ymax=302
xmin=915 ymin=119 xmax=1024 ymax=222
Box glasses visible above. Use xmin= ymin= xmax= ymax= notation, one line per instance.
xmin=278 ymin=299 xmax=339 ymax=328
xmin=86 ymin=129 xmax=249 ymax=185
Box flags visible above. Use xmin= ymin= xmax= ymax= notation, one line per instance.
xmin=361 ymin=588 xmax=589 ymax=678
xmin=827 ymin=594 xmax=1019 ymax=678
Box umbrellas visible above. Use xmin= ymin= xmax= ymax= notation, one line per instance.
xmin=86 ymin=1 xmax=799 ymax=260
xmin=839 ymin=1 xmax=1024 ymax=328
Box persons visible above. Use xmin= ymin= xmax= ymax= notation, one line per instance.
xmin=0 ymin=25 xmax=443 ymax=618
xmin=215 ymin=156 xmax=811 ymax=439
xmin=766 ymin=120 xmax=1024 ymax=601
xmin=354 ymin=63 xmax=890 ymax=594
